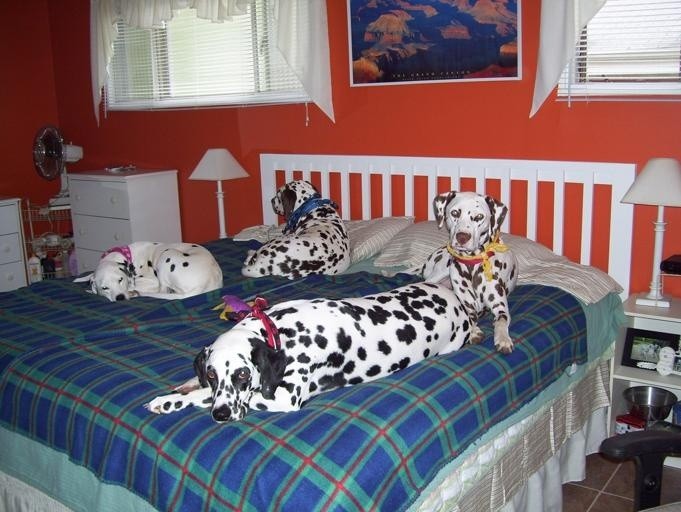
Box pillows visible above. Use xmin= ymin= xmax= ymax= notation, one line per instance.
xmin=233 ymin=215 xmax=414 ymax=267
xmin=373 ymin=217 xmax=573 ymax=279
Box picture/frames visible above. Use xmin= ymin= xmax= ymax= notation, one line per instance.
xmin=620 ymin=327 xmax=681 ymax=373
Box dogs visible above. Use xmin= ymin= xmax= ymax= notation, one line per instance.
xmin=420 ymin=189 xmax=520 ymax=356
xmin=70 ymin=240 xmax=224 ymax=304
xmin=237 ymin=179 xmax=353 ymax=279
xmin=141 ymin=279 xmax=473 ymax=426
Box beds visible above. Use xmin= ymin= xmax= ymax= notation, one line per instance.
xmin=0 ymin=153 xmax=637 ymax=512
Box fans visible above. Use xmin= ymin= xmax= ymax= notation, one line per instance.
xmin=32 ymin=124 xmax=83 ymax=206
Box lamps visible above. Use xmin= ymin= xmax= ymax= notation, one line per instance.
xmin=619 ymin=158 xmax=681 ymax=308
xmin=186 ymin=148 xmax=250 ymax=240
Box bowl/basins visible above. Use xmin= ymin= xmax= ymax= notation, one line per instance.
xmin=622 ymin=385 xmax=679 ymax=421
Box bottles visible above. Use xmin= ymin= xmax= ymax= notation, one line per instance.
xmin=28 ymin=252 xmax=44 ymax=284
xmin=62 ymin=249 xmax=70 ymax=278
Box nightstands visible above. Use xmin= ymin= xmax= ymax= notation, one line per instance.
xmin=606 ymin=292 xmax=680 ymax=470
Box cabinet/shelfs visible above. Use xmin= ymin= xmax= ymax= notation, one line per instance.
xmin=66 ymin=169 xmax=182 ymax=277
xmin=0 ymin=197 xmax=30 ymax=294
xmin=20 ymin=197 xmax=76 ymax=284
